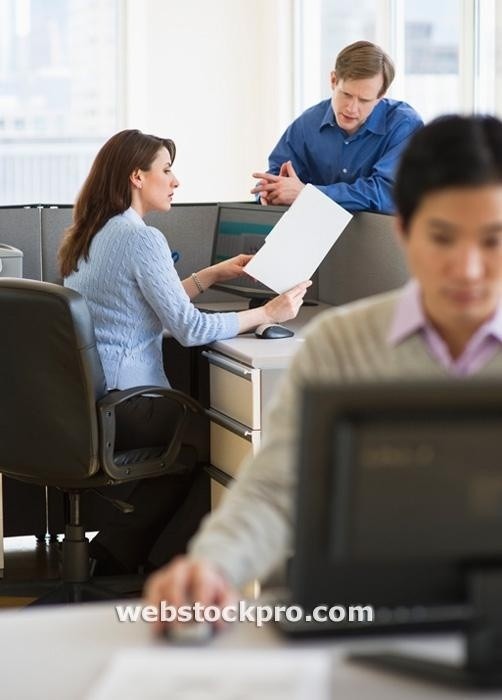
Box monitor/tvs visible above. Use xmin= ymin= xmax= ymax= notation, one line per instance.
xmin=205 ymin=202 xmax=320 ymax=309
xmin=289 ymin=376 xmax=502 ymax=688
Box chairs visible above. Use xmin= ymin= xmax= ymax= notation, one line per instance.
xmin=1 ymin=275 xmax=215 ymax=609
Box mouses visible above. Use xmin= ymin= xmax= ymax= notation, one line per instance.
xmin=170 ymin=606 xmax=220 ymax=649
xmin=255 ymin=323 xmax=294 ymax=339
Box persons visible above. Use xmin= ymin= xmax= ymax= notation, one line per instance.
xmin=141 ymin=114 xmax=502 ymax=635
xmin=250 ymin=40 xmax=425 ymax=217
xmin=57 ymin=130 xmax=313 ymax=577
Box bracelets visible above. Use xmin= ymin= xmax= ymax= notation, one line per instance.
xmin=192 ymin=273 xmax=204 ymax=293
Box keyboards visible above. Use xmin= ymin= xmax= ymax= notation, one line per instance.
xmin=264 ymin=596 xmax=471 ymax=641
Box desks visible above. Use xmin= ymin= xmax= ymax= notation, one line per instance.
xmin=148 ymin=291 xmax=345 ymax=517
xmin=2 ymin=591 xmax=501 ymax=700
